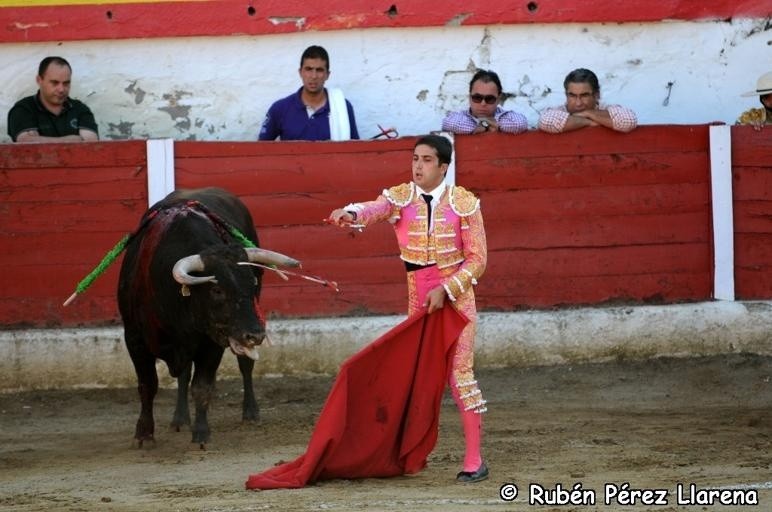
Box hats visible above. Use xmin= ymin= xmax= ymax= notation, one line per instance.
xmin=740 ymin=72 xmax=772 ymax=98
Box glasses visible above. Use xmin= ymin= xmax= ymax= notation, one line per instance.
xmin=469 ymin=93 xmax=497 ymax=104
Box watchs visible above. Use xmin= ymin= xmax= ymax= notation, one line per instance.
xmin=481 ymin=120 xmax=489 ymax=132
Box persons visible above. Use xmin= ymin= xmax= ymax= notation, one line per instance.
xmin=441 ymin=70 xmax=528 ymax=135
xmin=329 ymin=136 xmax=490 ymax=483
xmin=258 ymin=46 xmax=359 ymax=141
xmin=539 ymin=69 xmax=638 ymax=133
xmin=8 ymin=56 xmax=99 ymax=144
xmin=737 ymin=73 xmax=772 ymax=131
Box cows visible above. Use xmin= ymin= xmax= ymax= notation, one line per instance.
xmin=115 ymin=187 xmax=302 ymax=449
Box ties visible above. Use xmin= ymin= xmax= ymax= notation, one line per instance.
xmin=421 ymin=194 xmax=433 ymax=231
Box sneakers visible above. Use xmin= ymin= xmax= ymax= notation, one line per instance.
xmin=457 ymin=463 xmax=489 ymax=482
